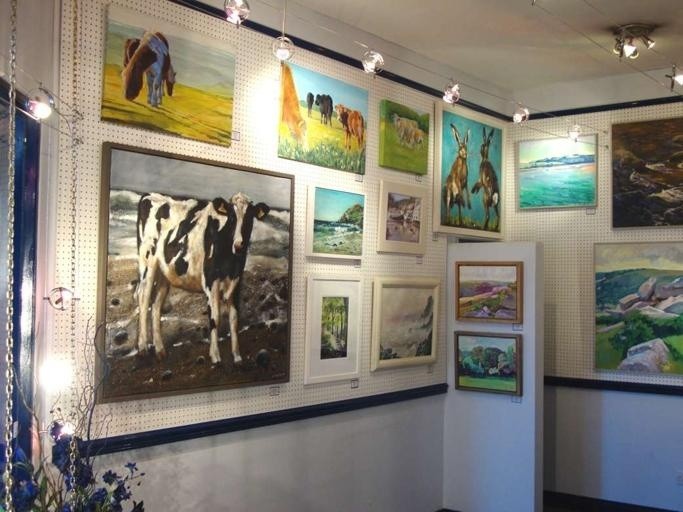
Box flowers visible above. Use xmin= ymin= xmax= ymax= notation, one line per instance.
xmin=0 ymin=427 xmax=147 ymax=512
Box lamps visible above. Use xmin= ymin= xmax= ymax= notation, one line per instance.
xmin=41 ymin=286 xmax=76 ymax=314
xmin=607 ymin=19 xmax=659 ymax=66
xmin=23 ymin=81 xmax=54 ymax=121
xmin=662 ymin=64 xmax=682 ymax=93
xmin=216 ymin=2 xmax=582 ymax=142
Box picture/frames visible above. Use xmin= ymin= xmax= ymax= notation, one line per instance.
xmin=375 ymin=178 xmax=431 ymax=256
xmin=453 ymin=330 xmax=523 ymax=398
xmin=607 ymin=116 xmax=683 ymax=231
xmin=453 ymin=259 xmax=523 ymax=325
xmin=591 ymin=238 xmax=683 ymax=374
xmin=514 ymin=133 xmax=599 ymax=209
xmin=370 ymin=272 xmax=441 ymax=371
xmin=431 ymin=97 xmax=507 ymax=241
xmin=303 ymin=273 xmax=364 ymax=387
xmin=90 ymin=134 xmax=297 ymax=409
xmin=303 ymin=179 xmax=365 ymax=263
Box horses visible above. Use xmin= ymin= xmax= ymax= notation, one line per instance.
xmin=123 ymin=38 xmax=177 ymax=96
xmin=119 ymin=31 xmax=171 ymax=107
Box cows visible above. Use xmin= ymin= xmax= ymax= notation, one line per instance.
xmin=282 ymin=62 xmax=310 ymax=153
xmin=315 ymin=94 xmax=333 ymax=128
xmin=335 ymin=104 xmax=364 ymax=151
xmin=307 ymin=92 xmax=314 ymax=118
xmin=137 ymin=191 xmax=271 ymax=368
xmin=390 ymin=113 xmax=423 ymax=152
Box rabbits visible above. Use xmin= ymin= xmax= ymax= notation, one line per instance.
xmin=443 ymin=123 xmax=472 ymax=227
xmin=470 ymin=126 xmax=500 ymax=230
